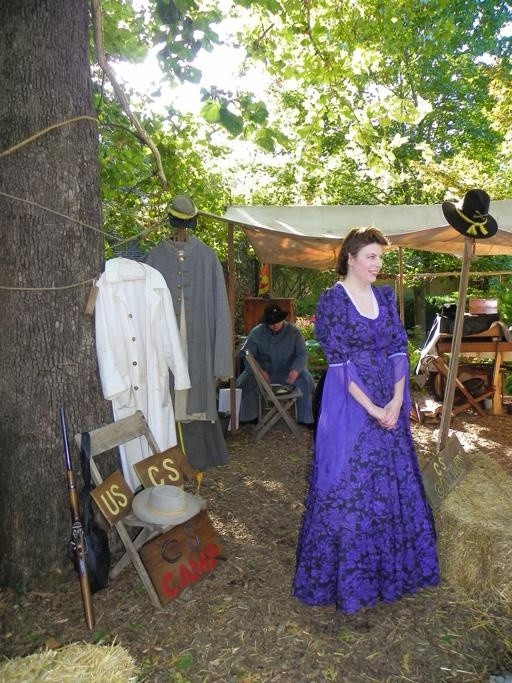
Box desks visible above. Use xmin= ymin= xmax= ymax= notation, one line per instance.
xmin=437 ymin=341 xmax=512 ymax=415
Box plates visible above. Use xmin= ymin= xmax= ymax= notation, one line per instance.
xmin=272 ymin=384 xmax=296 ymax=395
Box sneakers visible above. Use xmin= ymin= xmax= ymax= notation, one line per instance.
xmin=297 ymin=421 xmax=315 ymax=430
xmin=239 ymin=415 xmax=259 ymax=426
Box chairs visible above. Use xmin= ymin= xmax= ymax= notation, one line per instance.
xmin=74 ymin=410 xmax=208 ymax=609
xmin=428 ymin=352 xmax=497 ymax=420
xmin=244 ymin=349 xmax=304 ymax=443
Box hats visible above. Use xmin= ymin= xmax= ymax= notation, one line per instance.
xmin=463 ymin=377 xmax=485 ymax=399
xmin=131 ymin=484 xmax=201 ymax=526
xmin=441 ymin=188 xmax=499 ymax=240
xmin=435 ymin=303 xmax=457 ymax=321
xmin=258 ymin=303 xmax=289 ymax=325
xmin=168 ymin=195 xmax=201 ymax=229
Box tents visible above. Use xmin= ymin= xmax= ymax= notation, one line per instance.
xmin=197 ymin=197 xmax=512 ymax=429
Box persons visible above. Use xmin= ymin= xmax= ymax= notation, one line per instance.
xmin=232 ymin=304 xmax=317 ymax=432
xmin=291 ymin=225 xmax=443 ymax=616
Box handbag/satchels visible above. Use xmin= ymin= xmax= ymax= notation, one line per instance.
xmin=65 ymin=432 xmax=112 ymax=596
